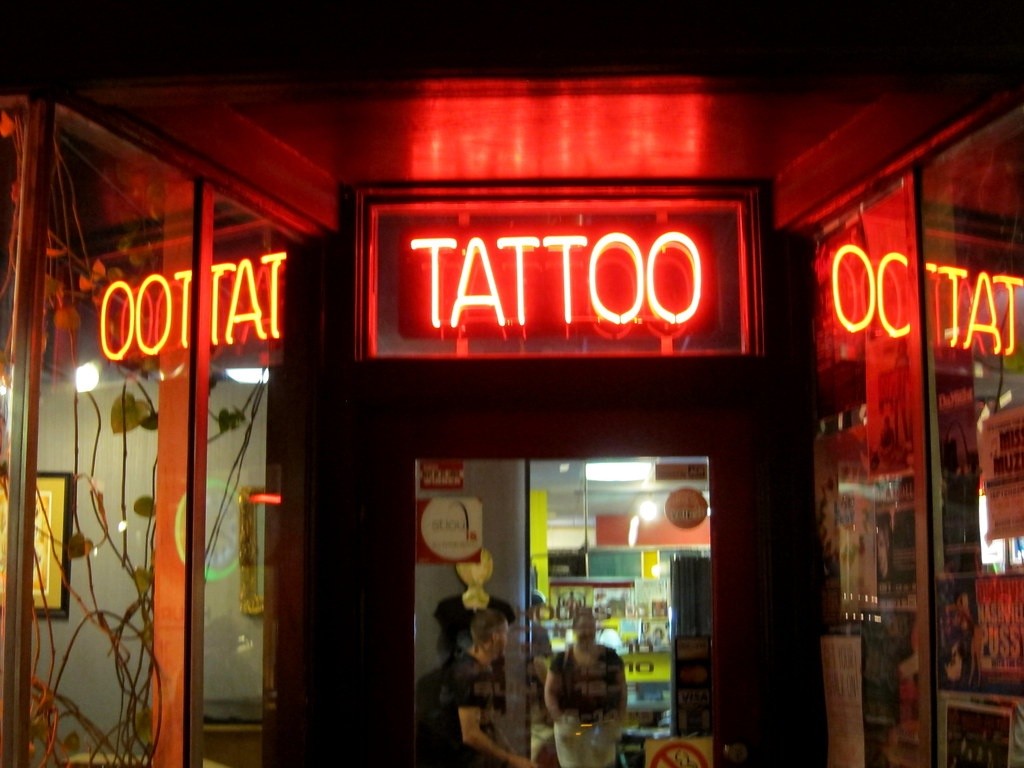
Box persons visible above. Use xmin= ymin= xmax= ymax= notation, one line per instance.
xmin=544 ymin=612 xmax=627 ymax=768
xmin=532 ymin=595 xmax=554 ymax=656
xmin=442 ymin=610 xmax=539 ymax=768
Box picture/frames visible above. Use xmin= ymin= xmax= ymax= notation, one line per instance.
xmin=0 ymin=472 xmax=74 ymax=620
